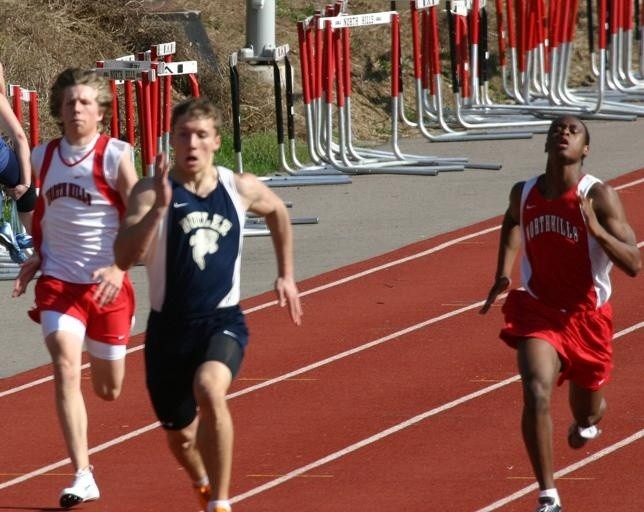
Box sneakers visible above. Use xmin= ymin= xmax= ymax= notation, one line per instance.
xmin=536 ymin=424 xmax=597 ymax=511
xmin=55 ymin=473 xmax=102 ymax=507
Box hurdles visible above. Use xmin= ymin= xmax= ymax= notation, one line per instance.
xmin=297 ymin=0 xmax=501 ymax=175
xmin=90 ymin=41 xmax=318 ymax=236
xmin=0 ymin=84 xmax=42 ymax=280
xmin=390 ymin=0 xmax=644 ymax=141
xmin=229 ymin=43 xmax=351 ymax=186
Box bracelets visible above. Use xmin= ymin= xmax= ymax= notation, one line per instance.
xmin=21 ymin=184 xmax=30 ymax=190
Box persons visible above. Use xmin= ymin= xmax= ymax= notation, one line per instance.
xmin=477 ymin=113 xmax=644 ymax=512
xmin=1 ymin=62 xmax=41 ymax=269
xmin=114 ymin=96 xmax=307 ymax=512
xmin=14 ymin=66 xmax=139 ymax=509
